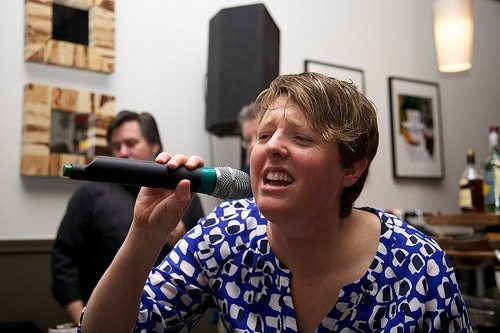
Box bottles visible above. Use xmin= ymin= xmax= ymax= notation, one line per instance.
xmin=458 ymin=149 xmax=485 ymax=213
xmin=482 ymin=127 xmax=500 ymax=214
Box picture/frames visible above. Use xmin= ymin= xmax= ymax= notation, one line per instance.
xmin=388 ymin=76 xmax=445 ymax=181
xmin=23 ymin=0 xmax=115 ymax=75
xmin=19 ymin=83 xmax=118 ymax=178
xmin=305 ymin=59 xmax=367 ymax=97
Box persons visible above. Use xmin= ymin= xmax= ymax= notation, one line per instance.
xmin=217 ymin=101 xmax=265 ymax=333
xmin=77 ymin=71 xmax=473 ymax=333
xmin=51 ymin=110 xmax=206 ymax=330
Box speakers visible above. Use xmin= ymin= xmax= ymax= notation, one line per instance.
xmin=205 ymin=4 xmax=279 ymax=136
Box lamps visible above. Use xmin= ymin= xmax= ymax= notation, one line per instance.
xmin=432 ymin=0 xmax=474 ymax=73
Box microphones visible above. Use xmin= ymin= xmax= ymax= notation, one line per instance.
xmin=62 ymin=156 xmax=251 ymax=200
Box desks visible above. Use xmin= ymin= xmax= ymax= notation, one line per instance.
xmin=420 ymin=208 xmax=500 ymax=333
